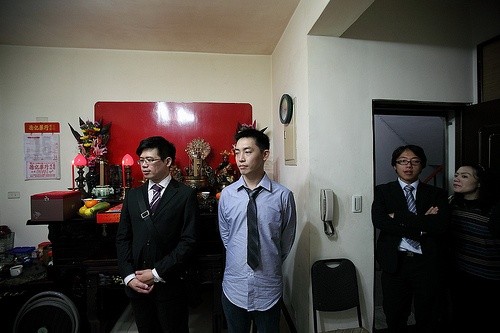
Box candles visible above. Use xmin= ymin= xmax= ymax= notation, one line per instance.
xmin=71 ymin=160 xmax=75 ymax=188
xmin=121 ymin=160 xmax=126 ymax=187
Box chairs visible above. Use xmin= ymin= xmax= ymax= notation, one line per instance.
xmin=12 ymin=291 xmax=80 ymax=333
xmin=311 ymin=258 xmax=371 ymax=333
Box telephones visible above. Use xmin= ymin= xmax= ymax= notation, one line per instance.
xmin=320 ymin=188 xmax=334 ymax=221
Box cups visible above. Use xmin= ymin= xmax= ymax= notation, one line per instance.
xmin=10 ymin=265 xmax=23 ymax=276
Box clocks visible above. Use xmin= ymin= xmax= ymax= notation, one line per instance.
xmin=279 ymin=94 xmax=294 ymax=124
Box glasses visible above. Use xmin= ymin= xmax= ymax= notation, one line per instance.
xmin=395 ymin=159 xmax=421 ymax=166
xmin=137 ymin=158 xmax=166 ymax=166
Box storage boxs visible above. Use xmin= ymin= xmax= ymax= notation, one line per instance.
xmin=30 ymin=191 xmax=81 ymax=222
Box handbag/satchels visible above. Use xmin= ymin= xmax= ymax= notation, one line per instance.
xmin=0 ymin=225 xmax=15 ymax=252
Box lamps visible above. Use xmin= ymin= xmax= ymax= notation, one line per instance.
xmin=73 ymin=153 xmax=87 ymax=194
xmin=121 ymin=154 xmax=134 ymax=188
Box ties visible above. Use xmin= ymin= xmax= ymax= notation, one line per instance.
xmin=403 ymin=185 xmax=420 ymax=250
xmin=241 ymin=185 xmax=263 ymax=272
xmin=149 ymin=183 xmax=164 ymax=213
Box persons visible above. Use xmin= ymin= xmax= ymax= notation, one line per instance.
xmin=218 ymin=127 xmax=296 ymax=333
xmin=371 ymin=146 xmax=453 ymax=333
xmin=448 ymin=164 xmax=500 ymax=333
xmin=117 ymin=136 xmax=205 ymax=333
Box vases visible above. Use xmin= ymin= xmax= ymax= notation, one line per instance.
xmin=86 ymin=165 xmax=100 ymax=197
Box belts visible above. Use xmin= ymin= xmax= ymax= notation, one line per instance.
xmin=406 ymin=251 xmax=423 ymax=256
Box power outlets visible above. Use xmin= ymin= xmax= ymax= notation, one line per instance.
xmin=8 ymin=191 xmax=20 ymax=198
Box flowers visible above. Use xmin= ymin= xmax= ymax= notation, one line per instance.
xmin=236 ymin=120 xmax=269 ymax=133
xmin=68 ymin=117 xmax=112 ymax=167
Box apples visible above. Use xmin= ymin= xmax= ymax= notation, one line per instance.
xmin=216 ymin=193 xmax=220 ymax=200
xmin=79 ymin=200 xmax=98 ymax=216
